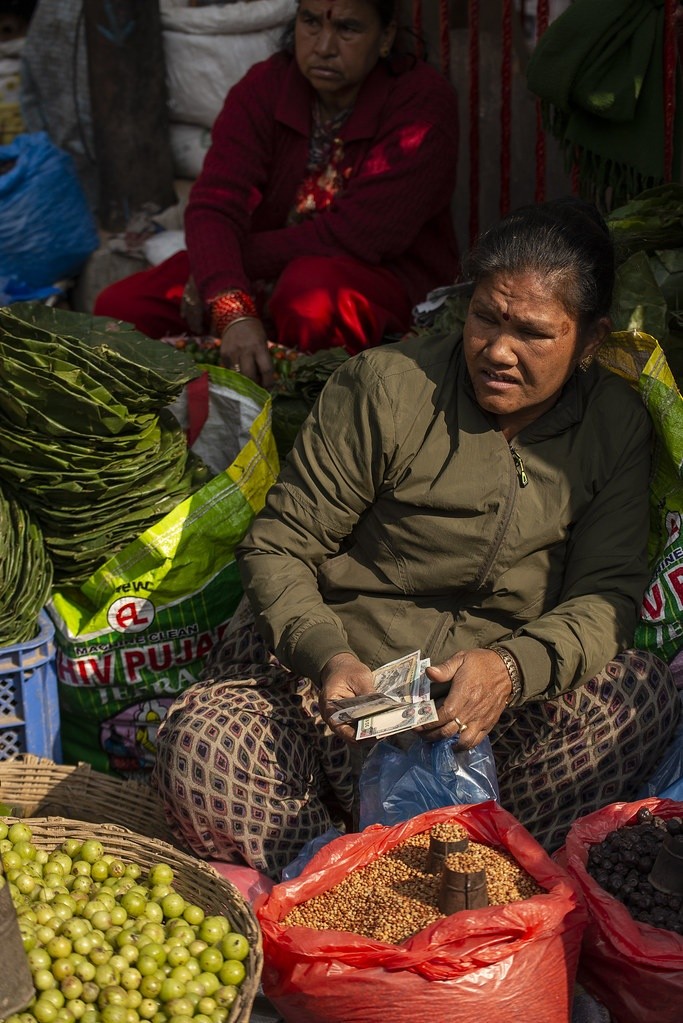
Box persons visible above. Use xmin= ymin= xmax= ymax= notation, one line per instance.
xmin=95 ymin=0 xmax=459 ymax=388
xmin=153 ymin=197 xmax=678 ymax=884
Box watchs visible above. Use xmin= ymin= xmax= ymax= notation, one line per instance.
xmin=480 ymin=644 xmax=523 ymax=709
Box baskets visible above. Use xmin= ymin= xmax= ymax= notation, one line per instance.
xmin=0 ymin=812 xmax=264 ymax=1023
xmin=0 ymin=752 xmax=172 ymax=840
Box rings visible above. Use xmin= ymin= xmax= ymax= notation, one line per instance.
xmin=454 ymin=717 xmax=467 ymax=734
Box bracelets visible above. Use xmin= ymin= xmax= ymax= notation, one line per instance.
xmin=220 ymin=316 xmax=260 ymax=339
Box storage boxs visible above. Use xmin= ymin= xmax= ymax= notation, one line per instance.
xmin=0 ymin=606 xmax=63 ymax=767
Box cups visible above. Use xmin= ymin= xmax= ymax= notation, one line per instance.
xmin=646 ymin=838 xmax=683 ymax=898
xmin=424 ymin=832 xmax=468 ymax=875
xmin=437 ymin=863 xmax=489 ymax=917
